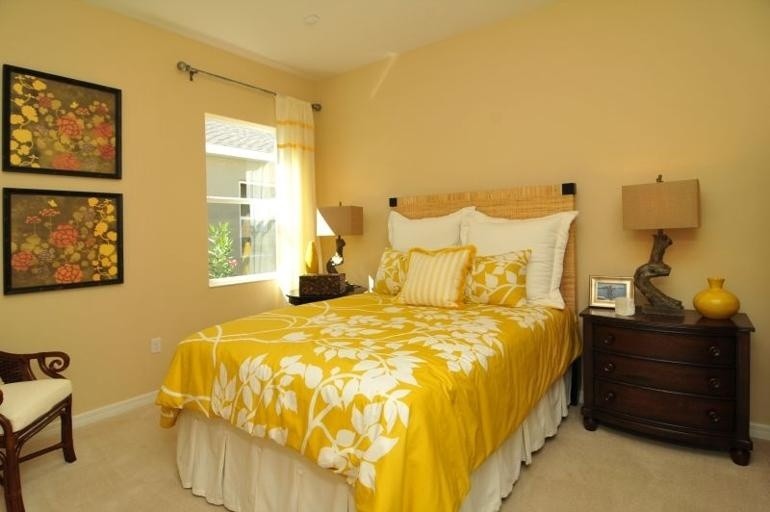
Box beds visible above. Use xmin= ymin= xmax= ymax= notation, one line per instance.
xmin=154 ymin=181 xmax=581 ymax=511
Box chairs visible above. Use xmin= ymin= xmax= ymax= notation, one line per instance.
xmin=1 ymin=341 xmax=80 ymax=511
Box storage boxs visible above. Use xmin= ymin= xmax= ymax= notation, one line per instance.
xmin=297 ymin=273 xmax=347 ymax=296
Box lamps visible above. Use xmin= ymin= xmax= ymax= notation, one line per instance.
xmin=316 ymin=199 xmax=363 ymax=272
xmin=621 ymin=173 xmax=701 ymax=316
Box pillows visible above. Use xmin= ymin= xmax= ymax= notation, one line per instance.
xmin=399 ymin=246 xmax=476 ymax=310
xmin=386 ymin=203 xmax=477 ymax=252
xmin=464 ymin=247 xmax=534 ymax=309
xmin=459 ymin=204 xmax=579 ymax=310
xmin=371 ymin=247 xmax=411 ymax=298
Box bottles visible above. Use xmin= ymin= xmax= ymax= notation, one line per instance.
xmin=692 ymin=275 xmax=741 ymax=320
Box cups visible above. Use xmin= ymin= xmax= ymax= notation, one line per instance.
xmin=614 ymin=296 xmax=635 ymax=316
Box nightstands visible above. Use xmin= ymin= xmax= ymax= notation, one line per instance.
xmin=577 ymin=306 xmax=755 ymax=466
xmin=285 ymin=283 xmax=368 ymax=307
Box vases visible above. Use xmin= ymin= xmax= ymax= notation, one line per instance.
xmin=691 ymin=276 xmax=739 ymax=319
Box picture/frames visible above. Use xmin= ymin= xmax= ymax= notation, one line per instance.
xmin=4 ymin=187 xmax=124 ymax=295
xmin=4 ymin=64 xmax=123 ymax=182
xmin=587 ymin=274 xmax=635 ymax=310
